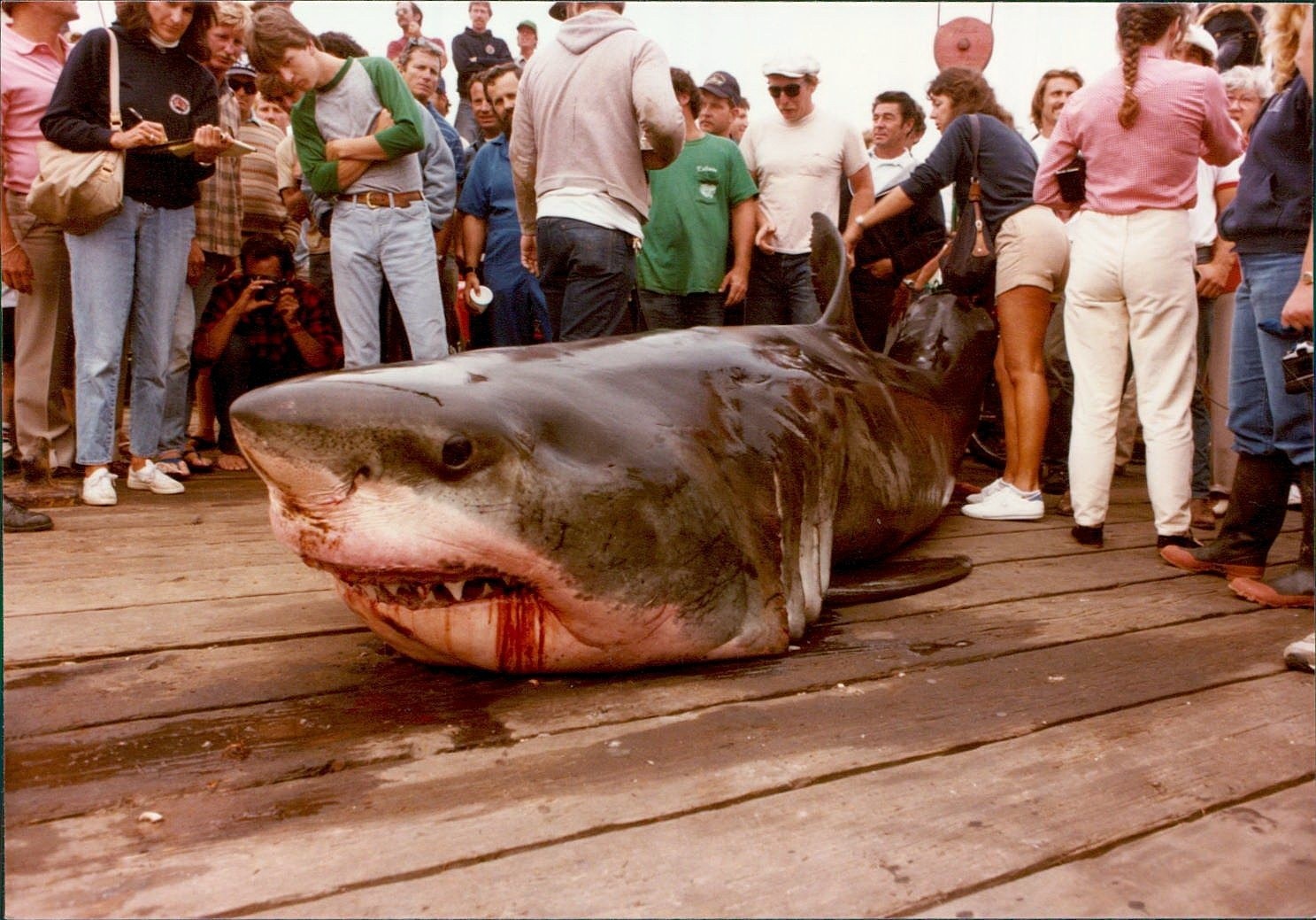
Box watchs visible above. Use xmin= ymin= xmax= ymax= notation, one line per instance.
xmin=1300 ymin=272 xmax=1313 ymax=284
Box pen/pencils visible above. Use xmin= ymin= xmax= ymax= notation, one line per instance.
xmin=126 ymin=106 xmax=143 ymax=123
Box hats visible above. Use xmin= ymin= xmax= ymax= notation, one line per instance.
xmin=516 ymin=20 xmax=536 ymax=30
xmin=408 ymin=37 xmax=444 ymax=56
xmin=224 ymin=53 xmax=256 ymax=77
xmin=549 ymin=1 xmax=624 ymax=21
xmin=764 ymin=57 xmax=820 ymax=78
xmin=698 ymin=71 xmax=740 ymax=105
xmin=1175 ymin=26 xmax=1219 ymax=62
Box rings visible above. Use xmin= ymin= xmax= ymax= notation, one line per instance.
xmin=150 ymin=135 xmax=154 ymax=141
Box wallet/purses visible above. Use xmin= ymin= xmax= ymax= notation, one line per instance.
xmin=1054 ymin=158 xmax=1087 ymax=202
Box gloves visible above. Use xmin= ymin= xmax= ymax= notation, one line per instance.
xmin=962 ymin=466 xmax=1203 ymax=548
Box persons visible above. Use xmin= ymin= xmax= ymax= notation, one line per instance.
xmin=0 ymin=0 xmax=294 ymax=534
xmin=234 ymin=0 xmax=1068 ymax=520
xmin=841 ymin=3 xmax=1314 ymax=676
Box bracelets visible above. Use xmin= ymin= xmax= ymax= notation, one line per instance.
xmin=463 ymin=267 xmax=480 ymax=275
xmin=853 ymin=217 xmax=864 ymax=229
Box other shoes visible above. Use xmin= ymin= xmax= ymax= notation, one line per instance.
xmin=1205 ymin=491 xmax=1230 ymax=517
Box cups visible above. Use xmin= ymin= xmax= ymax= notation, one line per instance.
xmin=470 ymin=286 xmax=493 ymax=313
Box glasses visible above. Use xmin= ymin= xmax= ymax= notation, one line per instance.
xmin=768 ymin=82 xmax=809 ymax=98
xmin=396 ymin=8 xmax=408 ymax=17
xmin=229 ymin=77 xmax=258 ymax=96
xmin=1225 ymin=92 xmax=1256 ymax=109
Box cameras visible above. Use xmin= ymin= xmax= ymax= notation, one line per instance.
xmin=257 ymin=277 xmax=288 ymax=303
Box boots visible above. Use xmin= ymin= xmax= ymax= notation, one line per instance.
xmin=1160 ymin=455 xmax=1294 ymax=582
xmin=1228 ymin=463 xmax=1316 ymax=608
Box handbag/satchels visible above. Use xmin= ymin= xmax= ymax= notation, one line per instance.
xmin=939 ymin=184 xmax=997 ymax=279
xmin=25 ymin=126 xmax=126 ymax=236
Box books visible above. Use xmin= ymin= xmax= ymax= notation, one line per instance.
xmin=144 ymin=134 xmax=257 ymax=158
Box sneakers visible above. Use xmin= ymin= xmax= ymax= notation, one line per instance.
xmin=1282 ymin=632 xmax=1316 ymax=670
xmin=0 ymin=459 xmax=184 ymax=534
xmin=1190 ymin=498 xmax=1216 ymax=529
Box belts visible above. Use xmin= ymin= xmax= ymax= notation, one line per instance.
xmin=335 ymin=191 xmax=422 ymax=208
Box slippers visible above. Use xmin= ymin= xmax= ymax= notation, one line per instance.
xmin=156 ymin=458 xmax=190 ymax=482
xmin=183 ymin=451 xmax=215 ymax=474
xmin=185 ymin=435 xmax=215 ymax=453
xmin=114 ymin=433 xmax=132 ymax=457
